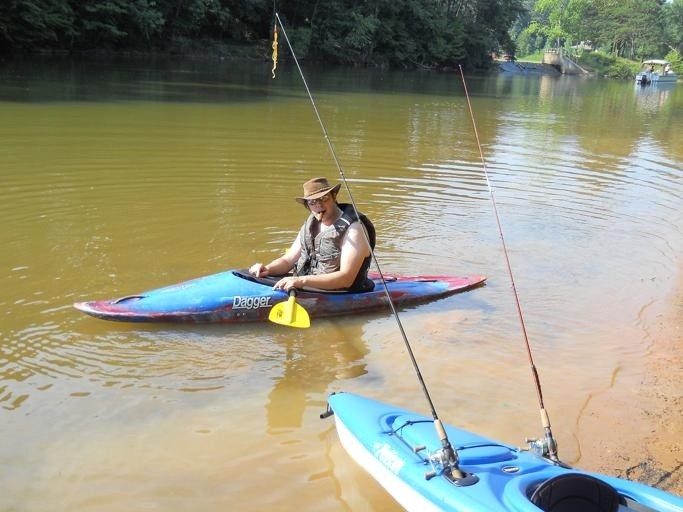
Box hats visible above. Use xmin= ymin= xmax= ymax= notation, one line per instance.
xmin=295 ymin=177 xmax=342 ymax=205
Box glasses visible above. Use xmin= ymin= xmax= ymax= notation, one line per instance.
xmin=306 ymin=193 xmax=330 ymax=205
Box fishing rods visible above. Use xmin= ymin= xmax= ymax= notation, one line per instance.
xmin=276 ymin=13 xmax=463 ymax=481
xmin=459 ymin=63 xmax=561 ymax=465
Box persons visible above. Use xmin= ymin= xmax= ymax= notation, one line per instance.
xmin=246 ymin=177 xmax=376 ymax=295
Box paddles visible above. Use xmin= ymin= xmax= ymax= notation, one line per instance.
xmin=268 ymin=264 xmax=310 ymax=328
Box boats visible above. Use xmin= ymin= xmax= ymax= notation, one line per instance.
xmin=636 ymin=60 xmax=678 ymax=84
xmin=320 ymin=392 xmax=683 ymax=512
xmin=73 ymin=269 xmax=488 ymax=323
